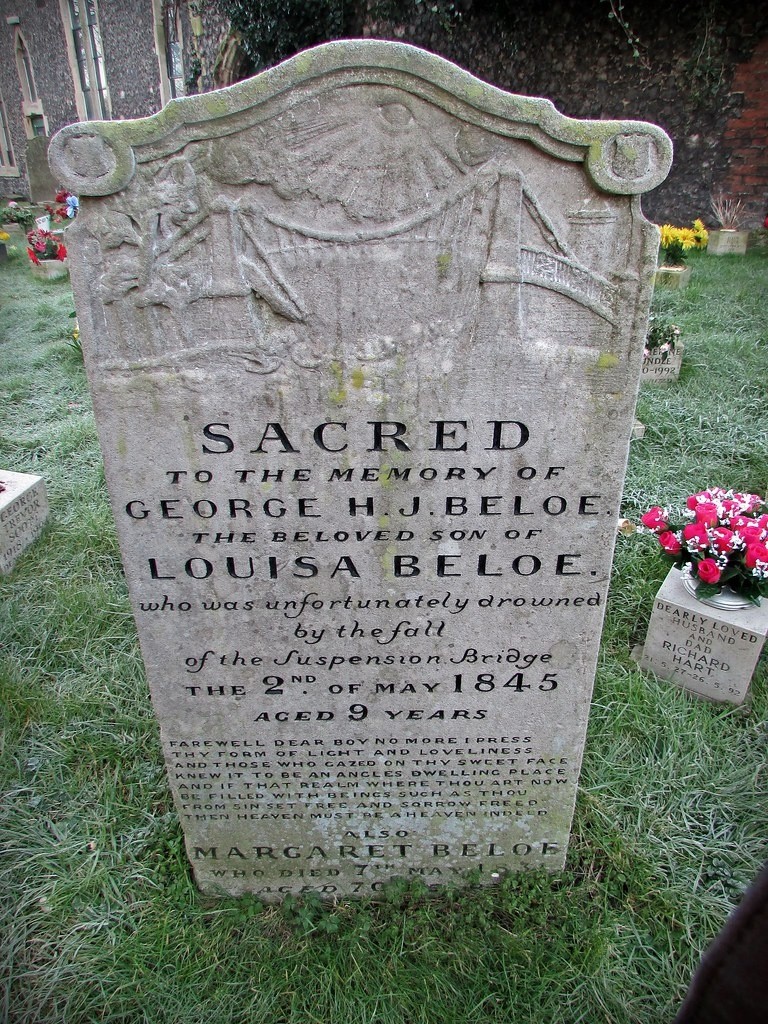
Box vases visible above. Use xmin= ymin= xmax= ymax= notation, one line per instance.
xmin=641 ymin=341 xmax=684 ymax=382
xmin=654 ymin=266 xmax=691 ymax=289
xmin=30 ymin=257 xmax=68 ymax=281
xmin=3 ymin=214 xmax=51 ymax=240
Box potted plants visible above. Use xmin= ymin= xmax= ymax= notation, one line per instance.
xmin=705 ymin=196 xmax=754 ymax=255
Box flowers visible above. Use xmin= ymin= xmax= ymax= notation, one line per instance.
xmin=659 ymin=218 xmax=710 ymax=269
xmin=643 ymin=486 xmax=768 ymax=607
xmin=67 ymin=312 xmax=83 ymax=362
xmin=644 ymin=316 xmax=679 ymax=364
xmin=26 ymin=227 xmax=67 ymax=267
xmin=43 ymin=186 xmax=78 ymax=222
xmin=0 ymin=200 xmax=38 ymax=246
